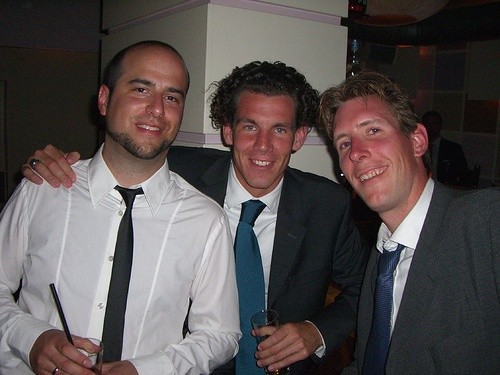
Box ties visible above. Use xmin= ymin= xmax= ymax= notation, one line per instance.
xmin=96 ymin=185 xmax=145 ymax=365
xmin=360 ymin=243 xmax=405 ymax=375
xmin=234 ymin=200 xmax=270 ymax=375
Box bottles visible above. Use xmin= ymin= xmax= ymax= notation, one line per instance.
xmin=346 ymin=40 xmax=364 ymax=80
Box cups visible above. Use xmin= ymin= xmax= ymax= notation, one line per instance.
xmin=251 ymin=309 xmax=290 ymax=375
xmin=74 ymin=337 xmax=104 ymax=375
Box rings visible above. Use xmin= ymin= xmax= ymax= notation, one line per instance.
xmin=30 ymin=159 xmax=40 ymax=169
xmin=52 ymin=366 xmax=59 ymax=375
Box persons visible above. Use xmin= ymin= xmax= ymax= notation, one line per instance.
xmin=318 ymin=70 xmax=500 ymax=375
xmin=20 ymin=61 xmax=368 ymax=375
xmin=0 ymin=39 xmax=245 ymax=375
